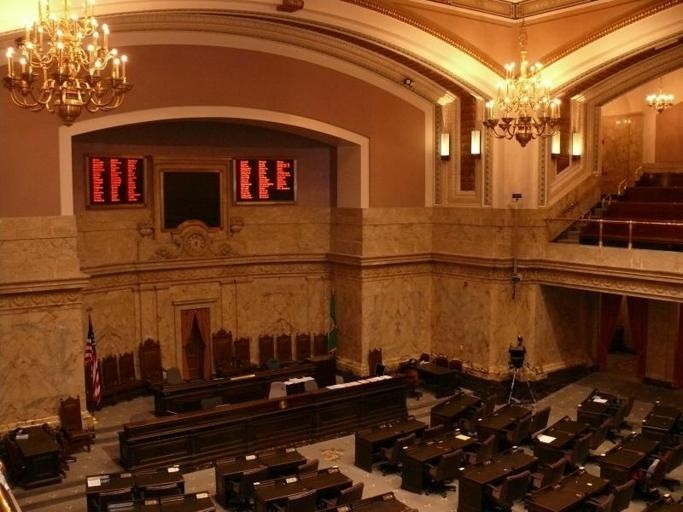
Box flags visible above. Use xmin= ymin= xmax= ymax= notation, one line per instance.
xmin=328 ymin=288 xmax=337 ymax=352
xmin=86 ymin=330 xmax=103 ymax=411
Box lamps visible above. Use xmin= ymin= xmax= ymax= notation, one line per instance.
xmin=572 ymin=131 xmax=583 ymax=158
xmin=551 ymin=129 xmax=561 ymax=154
xmin=469 ymin=129 xmax=481 ymax=157
xmin=645 ymin=72 xmax=675 ymax=113
xmin=2 ymin=0 xmax=134 ymax=127
xmin=482 ymin=0 xmax=564 ymax=148
xmin=439 ymin=132 xmax=450 ymax=159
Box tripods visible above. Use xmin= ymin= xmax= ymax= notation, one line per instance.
xmin=506 ymin=365 xmax=537 ymax=404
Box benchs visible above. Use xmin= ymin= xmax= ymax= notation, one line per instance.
xmin=577 ymin=165 xmax=682 ymax=251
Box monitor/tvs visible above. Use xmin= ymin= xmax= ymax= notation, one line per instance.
xmin=87 ymin=155 xmax=148 ymax=208
xmin=232 ymin=158 xmax=296 ymax=204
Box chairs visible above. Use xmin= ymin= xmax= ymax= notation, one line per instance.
xmin=370 ymin=347 xmax=467 ymax=400
xmin=356 ymin=392 xmax=682 ymax=512
xmin=84 ymin=448 xmax=419 ymax=512
xmin=59 ymin=396 xmax=93 ymax=453
xmin=0 ymin=425 xmax=76 ymax=489
xmin=86 ymin=329 xmax=338 ymax=410
